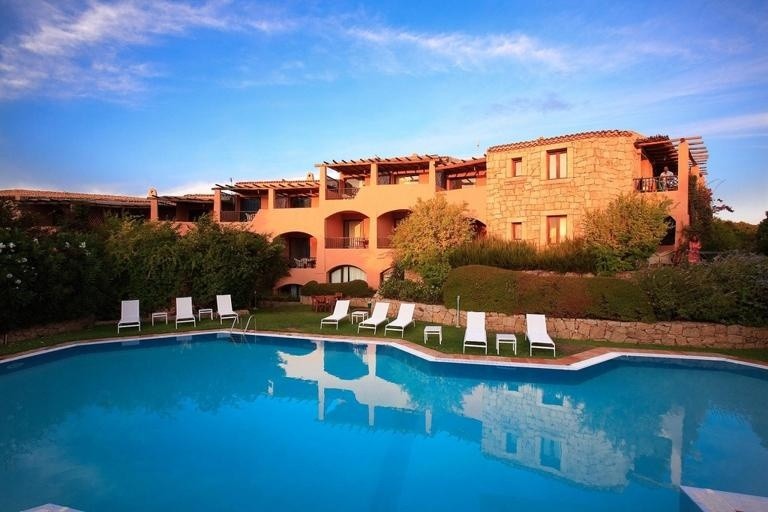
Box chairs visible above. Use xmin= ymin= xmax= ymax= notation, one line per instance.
xmin=525 ymin=313 xmax=556 ymax=358
xmin=321 ymin=300 xmax=351 ymax=329
xmin=117 ymin=299 xmax=141 ymax=334
xmin=311 ymin=292 xmax=351 ymax=313
xmin=358 ymin=302 xmax=390 ymax=335
xmin=175 ymin=297 xmax=196 ymax=329
xmin=385 ymin=304 xmax=415 ymax=338
xmin=463 ymin=312 xmax=488 ymax=355
xmin=216 ymin=294 xmax=239 ymax=325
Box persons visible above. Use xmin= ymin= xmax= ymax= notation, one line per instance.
xmin=660 ymin=167 xmax=673 ymax=192
xmin=688 ymin=234 xmax=703 ymax=266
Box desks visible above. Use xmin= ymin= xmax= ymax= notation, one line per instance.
xmin=495 ymin=333 xmax=517 ymax=356
xmin=423 ymin=325 xmax=442 ymax=345
xmin=352 ymin=311 xmax=368 ymax=325
xmin=198 ymin=309 xmax=213 ymax=321
xmin=152 ymin=313 xmax=168 ymax=326
